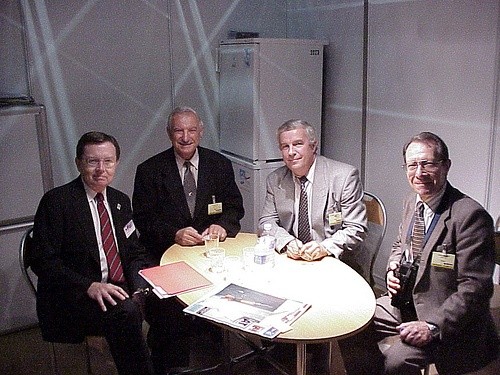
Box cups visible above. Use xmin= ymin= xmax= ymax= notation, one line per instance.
xmin=203 ymin=234 xmax=219 ymax=258
xmin=243 ymin=247 xmax=255 ymax=268
xmin=225 ymin=256 xmax=242 ymax=278
xmin=209 ymin=247 xmax=225 ymax=272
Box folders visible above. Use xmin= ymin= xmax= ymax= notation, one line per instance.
xmin=137 ymin=261 xmax=213 ymax=300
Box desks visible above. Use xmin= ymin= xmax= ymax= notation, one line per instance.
xmin=160 ymin=231 xmax=377 ymax=375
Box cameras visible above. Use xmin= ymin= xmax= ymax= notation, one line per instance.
xmin=390 ymin=262 xmax=420 ymax=308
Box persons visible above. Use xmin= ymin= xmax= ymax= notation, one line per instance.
xmin=337 ymin=132 xmax=498 ymax=375
xmin=33 ymin=133 xmax=189 ymax=375
xmin=132 ymin=106 xmax=245 ymax=265
xmin=257 ymin=119 xmax=369 ymax=275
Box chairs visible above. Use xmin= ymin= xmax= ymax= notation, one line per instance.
xmin=19 ymin=228 xmax=107 ymax=375
xmin=357 ymin=192 xmax=386 ymax=292
xmin=375 ymin=230 xmax=500 ymax=374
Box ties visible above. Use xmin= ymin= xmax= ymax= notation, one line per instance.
xmin=183 ymin=160 xmax=196 ymax=218
xmin=296 ymin=175 xmax=312 ymax=245
xmin=95 ymin=192 xmax=125 ymax=284
xmin=412 ymin=202 xmax=425 ymax=265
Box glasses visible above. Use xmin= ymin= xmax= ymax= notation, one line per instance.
xmin=79 ymin=157 xmax=117 ymax=167
xmin=407 ymin=158 xmax=447 ymax=170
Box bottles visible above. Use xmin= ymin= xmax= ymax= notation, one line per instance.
xmin=254 ymin=239 xmax=267 ymax=273
xmin=260 ymin=224 xmax=275 ymax=268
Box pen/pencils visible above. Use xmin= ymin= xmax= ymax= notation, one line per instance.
xmin=300 ymin=247 xmax=312 ymax=256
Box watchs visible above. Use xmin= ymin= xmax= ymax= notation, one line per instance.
xmin=427 ymin=323 xmax=439 ymax=340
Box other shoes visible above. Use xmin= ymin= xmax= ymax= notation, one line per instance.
xmin=209 ymin=325 xmax=225 ymax=345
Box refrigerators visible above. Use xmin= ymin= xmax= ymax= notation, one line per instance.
xmin=218 ymin=38 xmax=329 ymax=238
